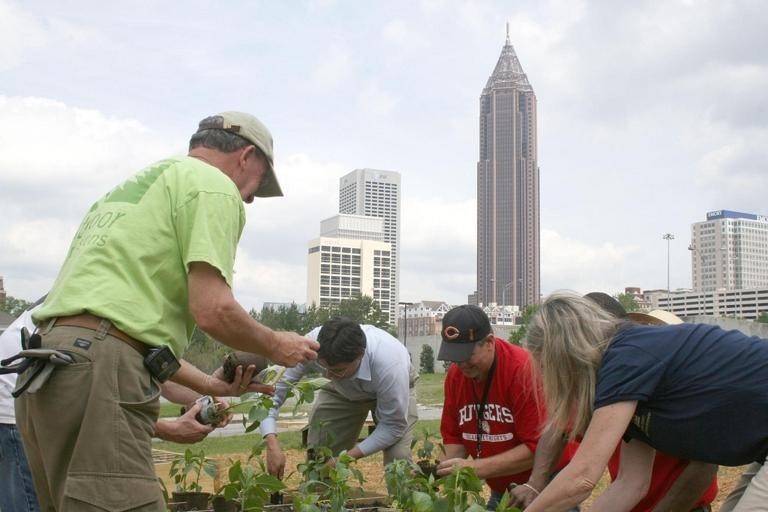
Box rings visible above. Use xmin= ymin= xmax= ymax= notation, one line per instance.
xmin=239 ymin=386 xmax=248 ymax=392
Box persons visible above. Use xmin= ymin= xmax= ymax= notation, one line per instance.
xmin=12 ymin=109 xmax=320 ymax=511
xmin=408 ymin=305 xmax=579 ymax=512
xmin=256 ymin=319 xmax=418 ymax=498
xmin=0 ymin=284 xmax=220 ymax=510
xmin=506 ymin=291 xmax=719 ymax=512
xmin=526 ymin=292 xmax=768 ymax=512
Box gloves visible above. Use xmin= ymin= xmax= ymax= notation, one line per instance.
xmin=1 ymin=326 xmax=74 ymax=398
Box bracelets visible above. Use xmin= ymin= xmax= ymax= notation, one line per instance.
xmin=523 ymin=483 xmax=542 ymax=497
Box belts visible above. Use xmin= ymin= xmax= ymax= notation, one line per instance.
xmin=52 ymin=314 xmax=150 ymax=358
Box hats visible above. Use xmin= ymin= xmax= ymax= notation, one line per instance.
xmin=196 ymin=109 xmax=284 ymax=198
xmin=436 ymin=304 xmax=491 ymax=362
xmin=626 ymin=308 xmax=685 ymax=326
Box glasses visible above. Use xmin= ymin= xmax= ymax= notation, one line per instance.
xmin=313 ymin=357 xmax=348 ymax=378
